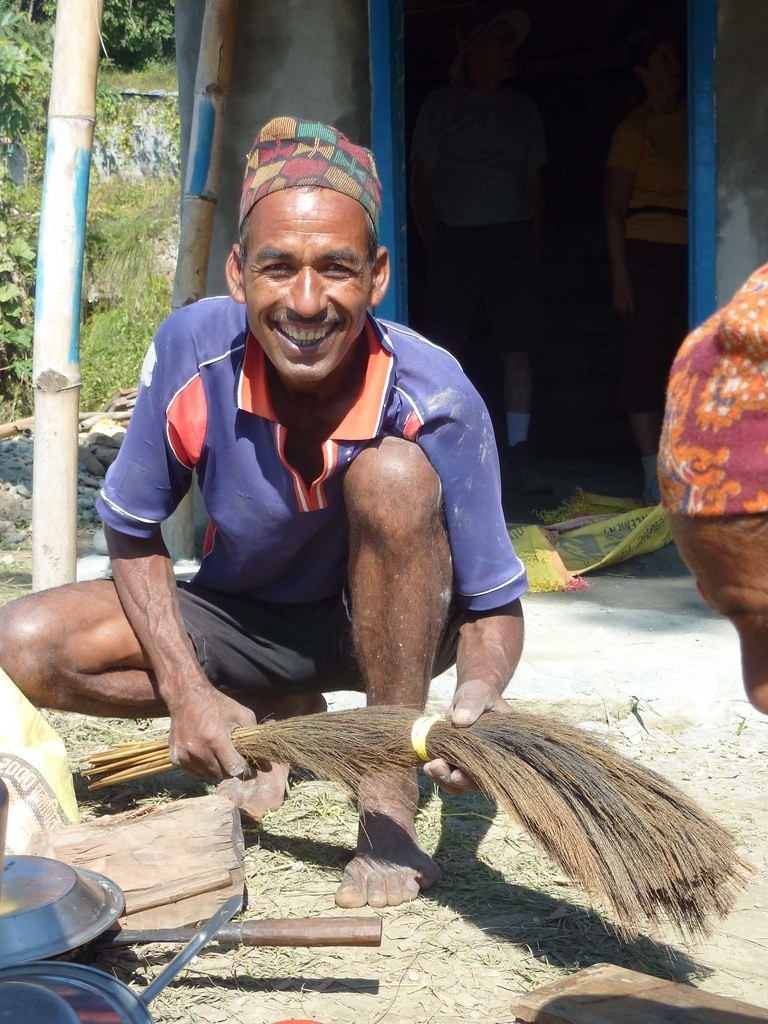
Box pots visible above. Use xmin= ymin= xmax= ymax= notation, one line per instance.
xmin=42 ymin=914 xmax=384 ymax=966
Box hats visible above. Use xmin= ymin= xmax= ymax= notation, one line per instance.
xmin=237 ymin=115 xmax=384 ymax=238
xmin=658 ymin=261 xmax=768 ymax=519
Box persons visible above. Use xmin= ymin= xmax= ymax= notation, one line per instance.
xmin=413 ymin=22 xmax=554 ymax=497
xmin=605 ymin=35 xmax=689 ymax=509
xmin=659 ymin=263 xmax=768 ymax=716
xmin=0 ymin=117 xmax=530 ymax=908
